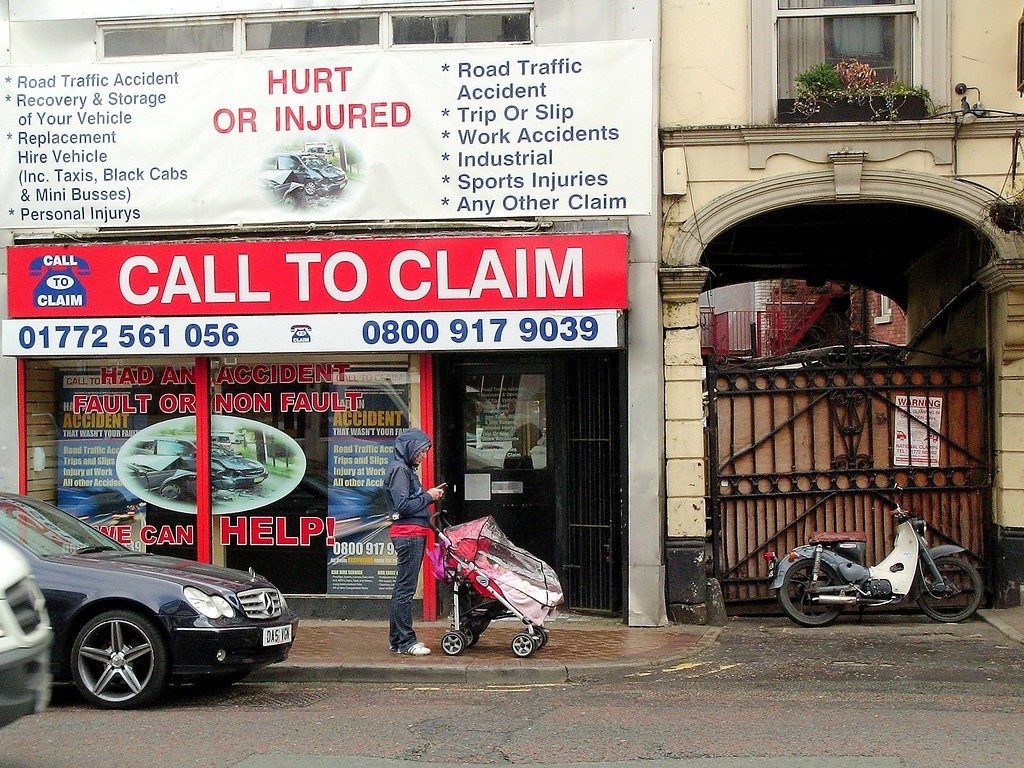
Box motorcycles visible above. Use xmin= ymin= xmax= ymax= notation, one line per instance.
xmin=764 ymin=483 xmax=984 ymax=628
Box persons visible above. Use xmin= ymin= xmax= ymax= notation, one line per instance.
xmin=383 ymin=428 xmax=444 ymax=656
xmin=503 ymin=423 xmax=543 ymax=469
xmin=475 ymin=553 xmax=563 ymax=626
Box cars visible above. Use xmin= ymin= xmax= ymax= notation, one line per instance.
xmin=125 ymin=431 xmax=269 ymax=500
xmin=0 ymin=491 xmax=299 ymax=729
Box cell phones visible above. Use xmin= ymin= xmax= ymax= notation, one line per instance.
xmin=442 ymin=485 xmax=449 ymax=490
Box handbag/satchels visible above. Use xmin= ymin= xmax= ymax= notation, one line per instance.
xmin=426 ymin=541 xmax=454 ymax=583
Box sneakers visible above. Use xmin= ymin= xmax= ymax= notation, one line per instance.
xmin=389 ymin=641 xmax=425 ymax=652
xmin=397 ymin=643 xmax=431 ymax=656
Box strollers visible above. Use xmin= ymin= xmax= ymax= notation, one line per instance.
xmin=430 ymin=510 xmax=549 ymax=658
